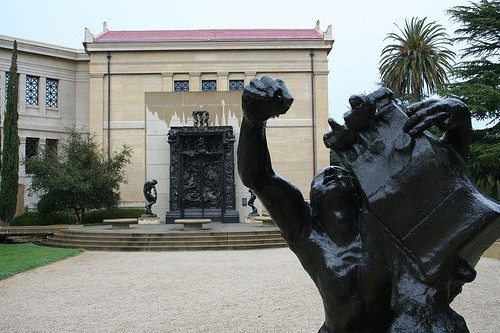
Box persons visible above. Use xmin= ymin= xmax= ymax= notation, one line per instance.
xmin=248 ymin=189 xmax=258 ymax=213
xmin=237 ymin=75 xmax=472 ymax=333
xmin=143 ymin=179 xmax=157 ymax=210
